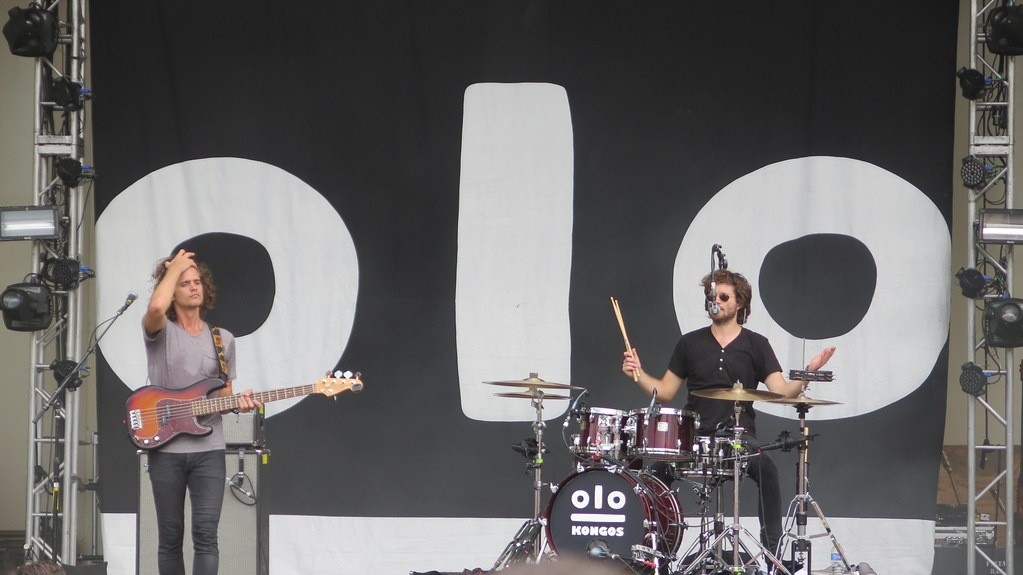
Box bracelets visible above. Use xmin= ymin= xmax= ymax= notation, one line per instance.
xmin=805 ymin=365 xmax=809 ymax=371
xmin=231 ymin=407 xmax=240 ymax=423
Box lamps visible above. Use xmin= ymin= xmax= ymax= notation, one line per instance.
xmin=52 ymin=78 xmax=82 ymax=105
xmin=985 ymin=6 xmax=1023 ymax=55
xmin=960 ymin=362 xmax=987 ymax=396
xmin=956 ymin=267 xmax=987 ymax=297
xmin=0 ymin=282 xmax=54 ymax=332
xmin=984 ymin=298 xmax=1023 ymax=347
xmin=2 ymin=3 xmax=59 ymax=58
xmin=54 ymin=259 xmax=81 ymax=287
xmin=50 ymin=358 xmax=82 ymax=391
xmin=55 ymin=156 xmax=82 ymax=187
xmin=957 ymin=66 xmax=986 ymax=98
xmin=961 ymin=155 xmax=988 ymax=189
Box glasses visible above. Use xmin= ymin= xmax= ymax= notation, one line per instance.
xmin=707 ymin=293 xmax=736 ymax=302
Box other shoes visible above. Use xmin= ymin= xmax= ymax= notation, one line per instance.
xmin=659 ymin=558 xmax=668 ymax=575
xmin=768 ymin=560 xmax=803 ymax=575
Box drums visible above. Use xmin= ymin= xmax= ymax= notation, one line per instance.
xmin=677 ymin=434 xmax=746 ymax=479
xmin=542 ymin=460 xmax=684 ymax=575
xmin=625 ymin=406 xmax=702 ymax=463
xmin=570 ymin=406 xmax=625 ymax=457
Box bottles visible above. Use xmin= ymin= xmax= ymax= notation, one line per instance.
xmin=830 ymin=544 xmax=844 ymax=575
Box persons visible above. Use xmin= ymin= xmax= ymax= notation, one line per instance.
xmin=142 ymin=249 xmax=262 ymax=575
xmin=624 ymin=269 xmax=835 ymax=575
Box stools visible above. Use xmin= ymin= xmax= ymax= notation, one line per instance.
xmin=676 ymin=468 xmax=763 ymax=575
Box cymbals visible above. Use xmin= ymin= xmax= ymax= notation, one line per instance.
xmin=691 ymin=388 xmax=786 ymax=402
xmin=482 ymin=378 xmax=585 ymax=391
xmin=769 ymin=395 xmax=843 ymax=407
xmin=490 ymin=389 xmax=573 ymax=402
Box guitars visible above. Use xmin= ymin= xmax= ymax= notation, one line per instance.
xmin=125 ymin=368 xmax=366 ymax=450
